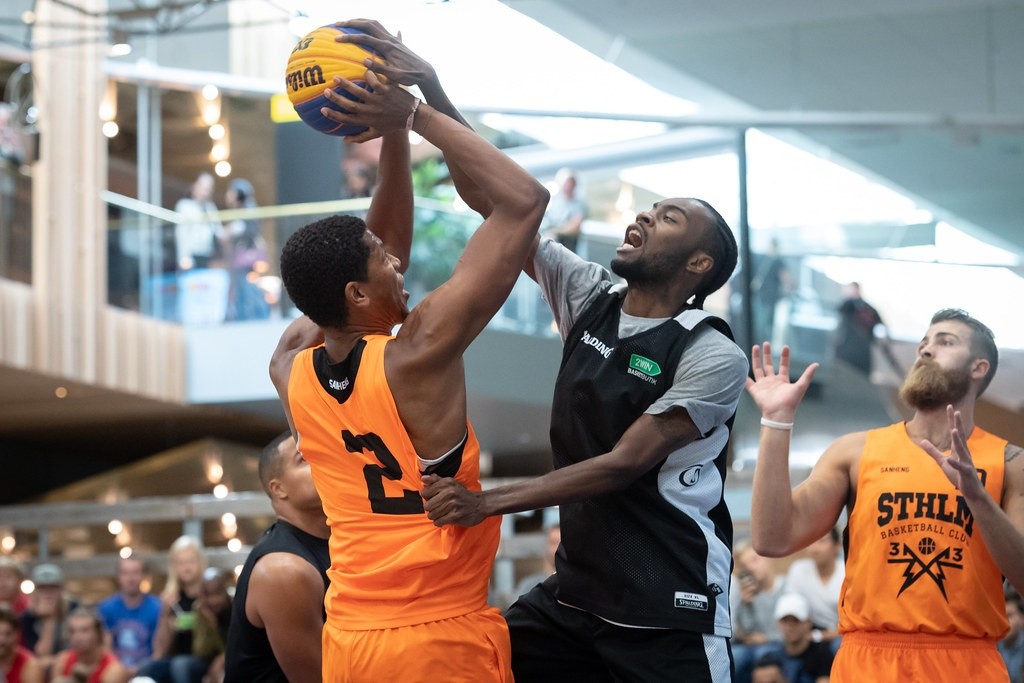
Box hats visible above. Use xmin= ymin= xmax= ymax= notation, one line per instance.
xmin=773 ymin=591 xmax=811 ymax=624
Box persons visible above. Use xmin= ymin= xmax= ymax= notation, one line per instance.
xmin=1 ymin=18 xmax=1024 ymax=683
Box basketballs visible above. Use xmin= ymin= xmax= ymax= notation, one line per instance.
xmin=283 ymin=21 xmax=395 ymax=139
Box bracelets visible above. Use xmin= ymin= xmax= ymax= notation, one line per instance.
xmin=419 ymin=108 xmax=435 ymax=136
xmin=760 ymin=417 xmax=794 ymax=430
xmin=406 ymin=97 xmax=421 ymax=130
xmin=812 ymin=629 xmax=824 ymax=642
